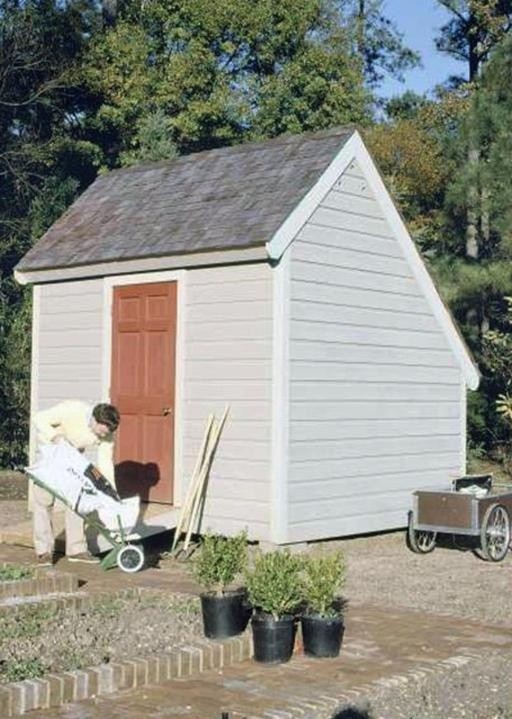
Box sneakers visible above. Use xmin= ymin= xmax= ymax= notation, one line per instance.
xmin=69 ymin=552 xmax=100 ymax=563
xmin=37 ymin=553 xmax=54 ymax=566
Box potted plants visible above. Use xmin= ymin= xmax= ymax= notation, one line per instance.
xmin=246 ymin=548 xmax=301 ymax=668
xmin=301 ymin=546 xmax=352 ymax=660
xmin=196 ymin=516 xmax=253 ymax=641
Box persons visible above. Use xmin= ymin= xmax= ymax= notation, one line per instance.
xmin=26 ymin=403 xmax=120 ymax=566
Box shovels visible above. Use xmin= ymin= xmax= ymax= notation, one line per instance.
xmin=173 ymin=406 xmax=230 ymax=562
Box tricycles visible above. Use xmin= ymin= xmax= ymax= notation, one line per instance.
xmin=408 ymin=480 xmax=512 ymax=562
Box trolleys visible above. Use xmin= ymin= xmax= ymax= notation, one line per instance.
xmin=11 ymin=435 xmax=146 ymax=574
